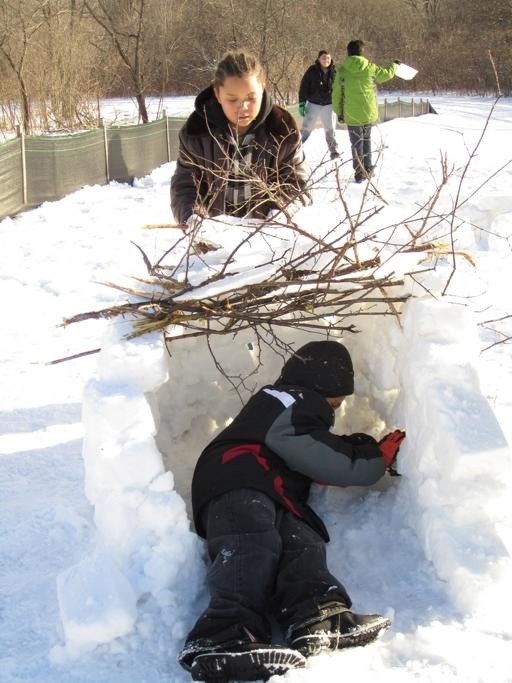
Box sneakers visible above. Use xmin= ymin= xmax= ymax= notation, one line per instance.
xmin=290 ymin=610 xmax=392 ymax=655
xmin=190 ymin=638 xmax=306 ymax=680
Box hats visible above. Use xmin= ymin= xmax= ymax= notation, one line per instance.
xmin=279 ymin=340 xmax=355 ymax=397
xmin=348 ymin=39 xmax=364 ymax=55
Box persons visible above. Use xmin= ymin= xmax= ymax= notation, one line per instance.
xmin=177 ymin=341 xmax=409 ymax=680
xmin=168 ymin=47 xmax=307 ymax=251
xmin=332 ymin=40 xmax=403 ymax=183
xmin=298 ymin=50 xmax=339 ymax=158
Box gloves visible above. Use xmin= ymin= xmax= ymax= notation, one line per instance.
xmin=298 ymin=103 xmax=308 ymax=118
xmin=338 ymin=116 xmax=345 ymax=124
xmin=374 ymin=428 xmax=405 ymax=470
